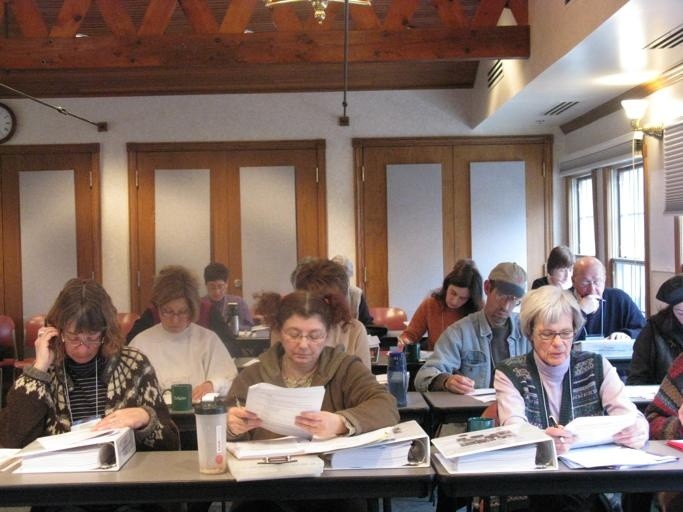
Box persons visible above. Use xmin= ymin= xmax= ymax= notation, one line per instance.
xmin=569 ymin=257 xmax=647 ymax=341
xmin=198 ymin=263 xmax=251 ymax=330
xmin=644 ymin=347 xmax=683 ymax=439
xmin=399 ymin=258 xmax=489 ymax=350
xmin=252 ymin=293 xmax=285 ymax=323
xmin=532 ymin=246 xmax=581 ymax=293
xmin=128 ymin=267 xmax=238 ymax=405
xmin=493 ymin=286 xmax=649 ymax=453
xmin=415 ymin=263 xmax=533 ymax=393
xmin=628 ymin=275 xmax=683 ymax=385
xmin=0 ymin=278 xmax=181 ymax=457
xmin=227 ymin=292 xmax=400 ymax=441
xmin=272 ymin=259 xmax=370 ymax=372
xmin=330 ymin=255 xmax=373 ymax=331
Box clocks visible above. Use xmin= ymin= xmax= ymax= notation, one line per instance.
xmin=0 ymin=104 xmax=17 ymax=144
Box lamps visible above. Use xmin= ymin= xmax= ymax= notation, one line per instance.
xmin=494 ymin=6 xmax=519 ymax=27
xmin=622 ymin=98 xmax=662 ymax=138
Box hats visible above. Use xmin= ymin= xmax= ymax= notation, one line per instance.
xmin=655 ymin=277 xmax=683 ymax=304
xmin=331 ymin=253 xmax=353 ymax=278
xmin=489 ymin=262 xmax=528 ymax=298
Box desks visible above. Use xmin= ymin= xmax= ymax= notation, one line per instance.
xmin=167 ymin=391 xmax=429 ymax=431
xmin=0 ymin=449 xmax=436 ymax=512
xmin=429 ymin=441 xmax=682 ymax=512
xmin=235 ymin=350 xmax=434 ymax=375
xmin=423 ymin=384 xmax=659 ymax=413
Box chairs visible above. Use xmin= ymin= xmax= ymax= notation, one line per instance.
xmin=0 ymin=316 xmax=18 ymax=383
xmin=13 ymin=317 xmax=45 ymax=370
xmin=115 ymin=313 xmax=140 ymax=337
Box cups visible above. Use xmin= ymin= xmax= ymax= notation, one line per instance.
xmin=162 ymin=384 xmax=192 ymax=412
xmin=467 ymin=417 xmax=495 ymax=432
xmin=192 ymin=401 xmax=227 ymax=474
xmin=405 ymin=343 xmax=420 ymax=360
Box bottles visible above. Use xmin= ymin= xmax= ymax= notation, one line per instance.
xmin=387 ymin=351 xmax=408 ymax=406
xmin=228 ymin=306 xmax=240 ymax=336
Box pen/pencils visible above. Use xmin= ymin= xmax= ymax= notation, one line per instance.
xmin=451 ymin=366 xmax=466 ymax=377
xmin=44 ymin=317 xmax=48 ymax=327
xmin=233 ymin=393 xmax=249 ymax=425
xmin=548 ymin=414 xmax=566 ymax=445
xmin=397 ymin=335 xmax=405 ymax=346
xmin=585 ymin=292 xmax=606 ymax=302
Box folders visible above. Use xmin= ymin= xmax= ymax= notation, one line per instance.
xmin=323 ymin=419 xmax=432 ymax=472
xmin=10 ymin=426 xmax=137 ymax=475
xmin=571 ymin=339 xmax=636 ymax=356
xmin=431 ymin=421 xmax=559 ymax=476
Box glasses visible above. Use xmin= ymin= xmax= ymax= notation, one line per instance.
xmin=156 ymin=301 xmax=192 ymax=321
xmin=573 ymin=277 xmax=604 ymax=289
xmin=489 ymin=289 xmax=521 ymax=307
xmin=530 ymin=326 xmax=576 ymax=339
xmin=281 ymin=325 xmax=326 ymax=344
xmin=205 ymin=283 xmax=226 ymax=291
xmin=59 ymin=329 xmax=106 ymax=348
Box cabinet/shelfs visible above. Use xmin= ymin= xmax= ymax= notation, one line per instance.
xmin=126 ymin=140 xmax=327 ymax=330
xmin=353 ymin=133 xmax=554 ymax=312
xmin=0 ymin=144 xmax=102 ymax=358
